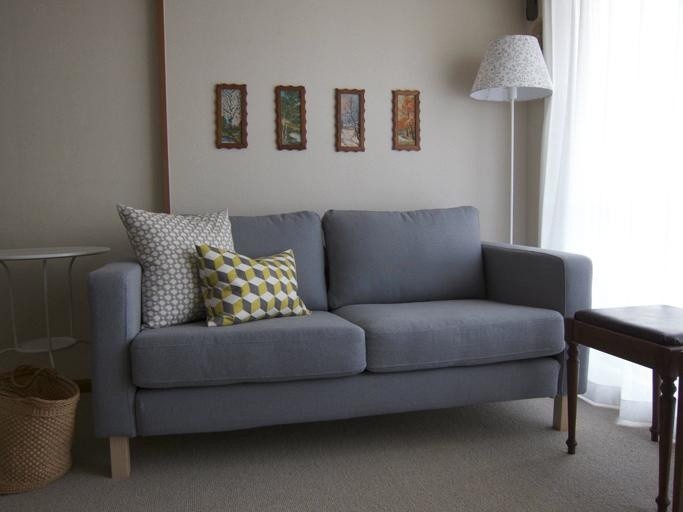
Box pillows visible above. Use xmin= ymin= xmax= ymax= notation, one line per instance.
xmin=195 ymin=242 xmax=312 ymax=328
xmin=116 ymin=202 xmax=236 ymax=328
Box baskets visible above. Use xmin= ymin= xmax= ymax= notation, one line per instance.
xmin=0 ymin=365 xmax=79 ymax=495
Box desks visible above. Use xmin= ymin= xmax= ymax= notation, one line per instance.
xmin=0 ymin=245 xmax=114 ymax=374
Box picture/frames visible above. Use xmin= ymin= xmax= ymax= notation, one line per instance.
xmin=273 ymin=84 xmax=308 ymax=151
xmin=213 ymin=82 xmax=251 ymax=150
xmin=390 ymin=89 xmax=422 ymax=154
xmin=333 ymin=87 xmax=366 ymax=152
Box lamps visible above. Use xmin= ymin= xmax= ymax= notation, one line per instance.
xmin=468 ymin=32 xmax=554 ymax=244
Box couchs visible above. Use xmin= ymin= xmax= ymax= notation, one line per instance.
xmin=82 ymin=204 xmax=594 ymax=482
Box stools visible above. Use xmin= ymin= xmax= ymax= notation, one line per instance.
xmin=561 ymin=303 xmax=682 ymax=511
